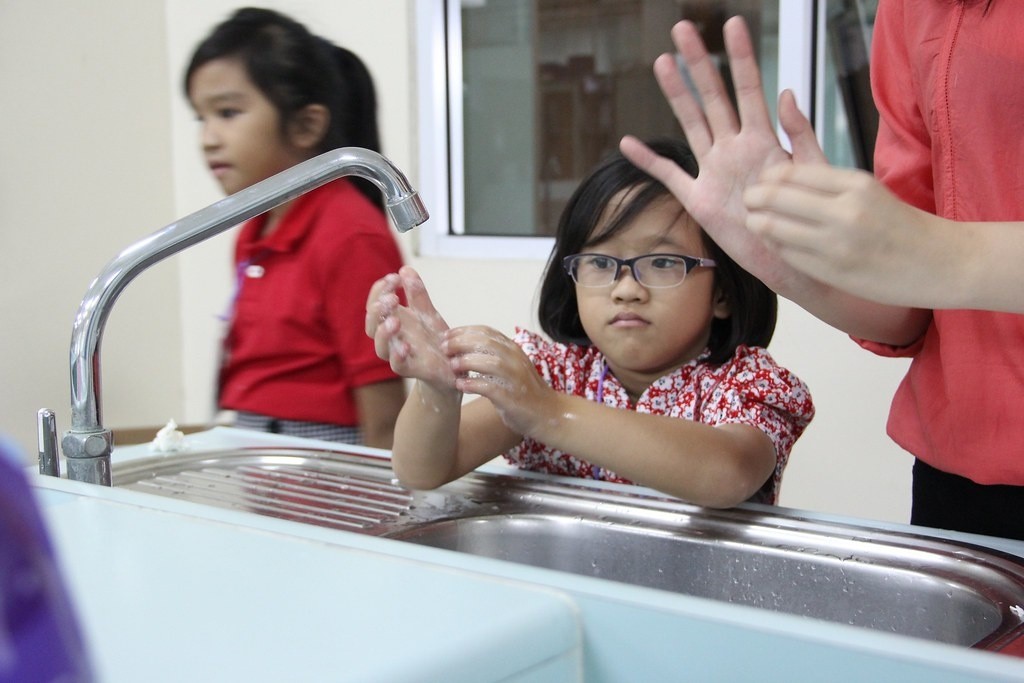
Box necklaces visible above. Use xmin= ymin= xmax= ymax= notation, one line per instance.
xmin=593 ymin=366 xmax=609 ymax=481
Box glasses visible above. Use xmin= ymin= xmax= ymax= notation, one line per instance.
xmin=560 ymin=253 xmax=719 ymax=289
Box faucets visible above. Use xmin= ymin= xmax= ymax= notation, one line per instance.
xmin=61 ymin=145 xmax=429 ymax=488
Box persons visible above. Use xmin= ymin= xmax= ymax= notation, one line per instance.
xmin=365 ymin=137 xmax=815 ymax=510
xmin=619 ymin=0 xmax=1024 ymax=541
xmin=184 ymin=7 xmax=411 ymax=450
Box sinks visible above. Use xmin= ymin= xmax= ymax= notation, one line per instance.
xmin=364 ymin=501 xmax=1024 ymax=652
